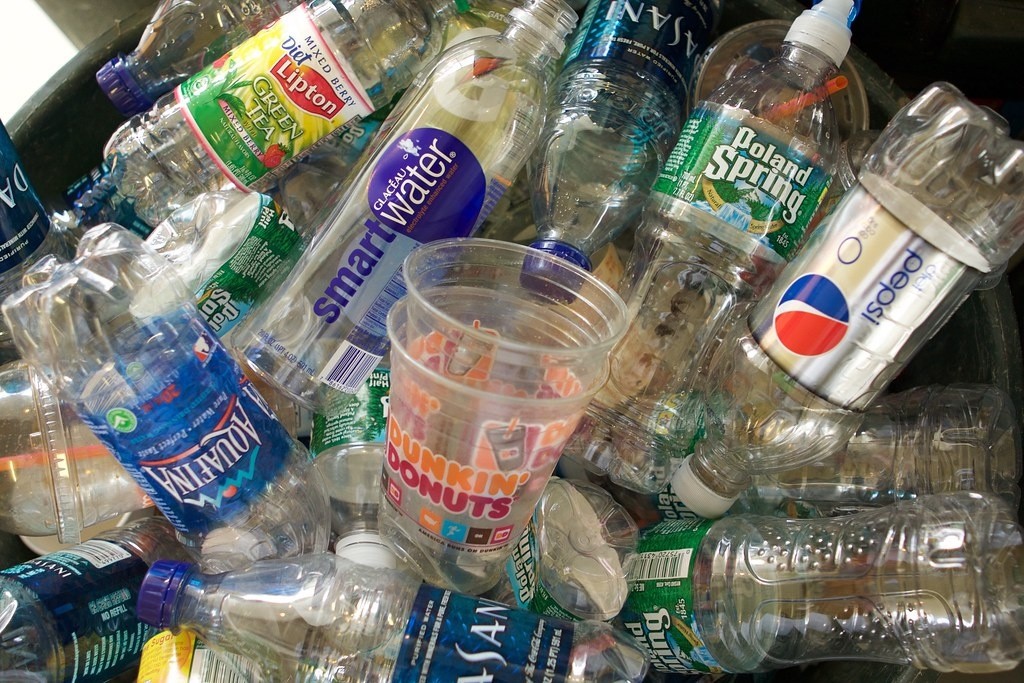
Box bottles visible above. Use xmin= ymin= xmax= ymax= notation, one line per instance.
xmin=0 ymin=0 xmax=1024 ymax=683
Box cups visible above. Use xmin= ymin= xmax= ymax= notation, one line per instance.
xmin=401 ymin=236 xmax=633 ymax=397
xmin=375 ymin=287 xmax=610 ymax=597
